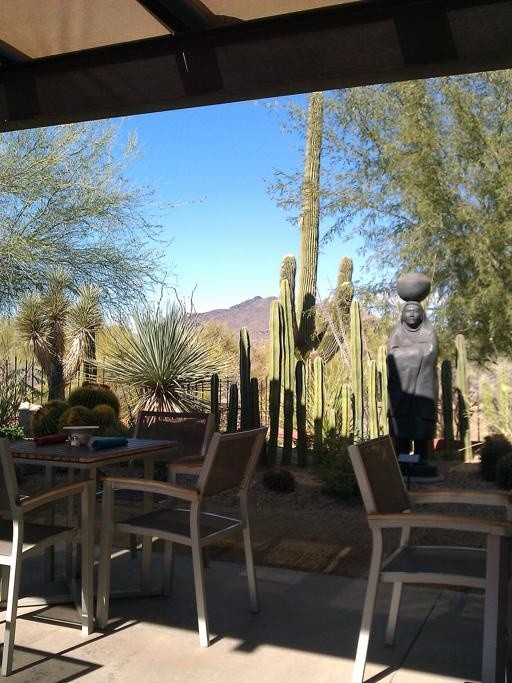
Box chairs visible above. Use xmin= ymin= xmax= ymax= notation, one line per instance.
xmin=130 ymin=410 xmax=211 ymax=487
xmin=0 ymin=437 xmax=96 ymax=677
xmin=97 ymin=423 xmax=269 ymax=649
xmin=347 ymin=433 xmax=512 ymax=683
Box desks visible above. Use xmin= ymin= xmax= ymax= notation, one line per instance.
xmin=4 ymin=427 xmax=175 ymax=523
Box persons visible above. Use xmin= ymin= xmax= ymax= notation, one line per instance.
xmin=384 ymin=300 xmax=440 ymax=478
